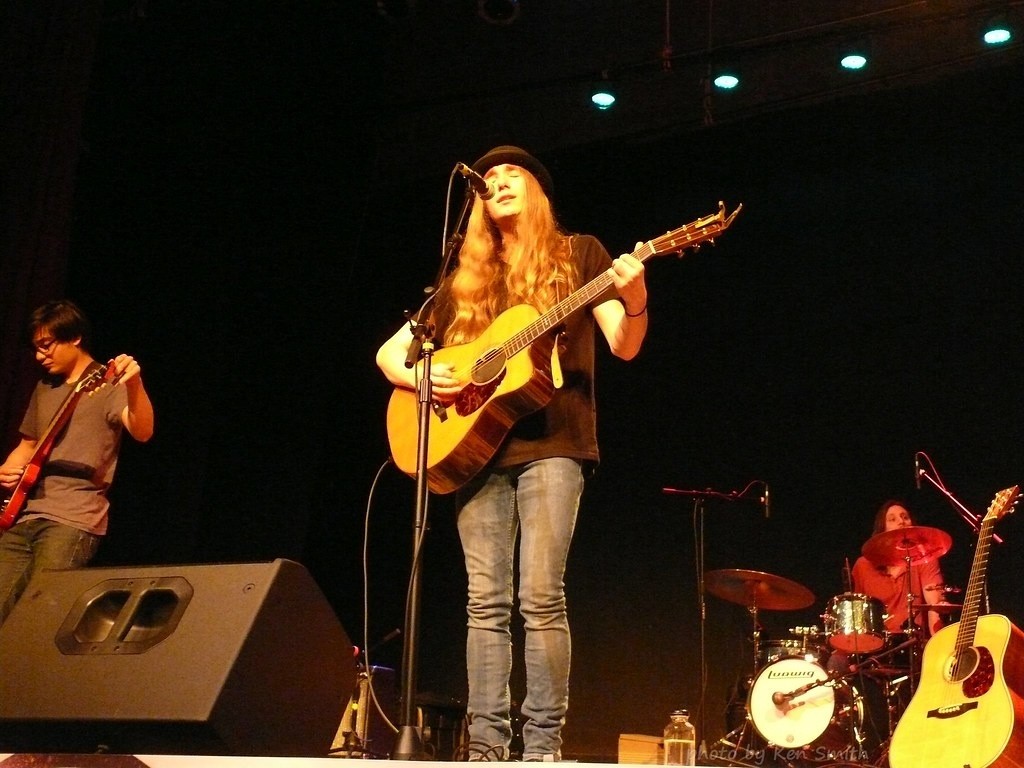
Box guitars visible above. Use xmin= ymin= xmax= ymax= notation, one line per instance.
xmin=887 ymin=486 xmax=1024 ymax=768
xmin=383 ymin=197 xmax=744 ymax=494
xmin=1 ymin=359 xmax=125 ymax=530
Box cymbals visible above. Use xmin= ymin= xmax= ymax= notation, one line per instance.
xmin=704 ymin=569 xmax=816 ymax=610
xmin=861 ymin=526 xmax=953 ymax=566
xmin=905 ymin=603 xmax=963 ymax=612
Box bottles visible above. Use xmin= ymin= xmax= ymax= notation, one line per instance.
xmin=665 ymin=709 xmax=695 ymax=766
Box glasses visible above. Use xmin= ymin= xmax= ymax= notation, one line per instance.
xmin=35 ymin=338 xmax=57 ymax=354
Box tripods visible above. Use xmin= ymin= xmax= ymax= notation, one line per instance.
xmin=695 ymin=579 xmax=795 ymax=768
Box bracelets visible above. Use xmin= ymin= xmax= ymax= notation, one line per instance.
xmin=625 ymin=305 xmax=647 ymax=318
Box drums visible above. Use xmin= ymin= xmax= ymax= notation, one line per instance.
xmin=746 ymin=658 xmax=865 ymax=762
xmin=822 ymin=591 xmax=890 ymax=654
xmin=754 ymin=635 xmax=832 ymax=666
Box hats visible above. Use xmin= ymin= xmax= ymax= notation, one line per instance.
xmin=470 ymin=146 xmax=554 ymax=195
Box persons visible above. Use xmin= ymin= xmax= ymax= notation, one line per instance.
xmin=0 ymin=300 xmax=154 ymax=630
xmin=377 ymin=146 xmax=742 ymax=762
xmin=851 ymin=501 xmax=950 ymax=639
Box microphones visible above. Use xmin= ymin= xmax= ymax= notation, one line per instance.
xmin=458 ymin=163 xmax=495 ymax=200
xmin=915 ymin=454 xmax=921 ymax=490
xmin=765 ymin=485 xmax=769 ymax=518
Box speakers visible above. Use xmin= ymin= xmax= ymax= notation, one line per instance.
xmin=0 ymin=558 xmax=358 ymax=757
